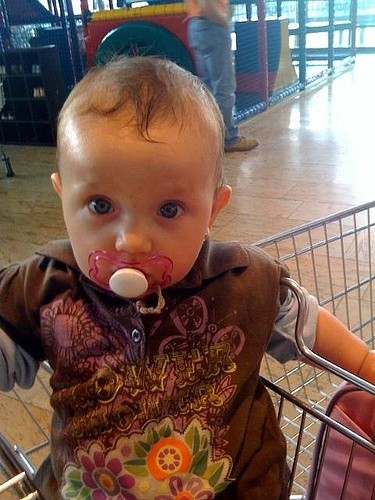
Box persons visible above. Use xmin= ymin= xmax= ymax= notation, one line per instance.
xmin=1 ymin=54 xmax=375 ymax=500
xmin=182 ymin=0 xmax=259 ymax=153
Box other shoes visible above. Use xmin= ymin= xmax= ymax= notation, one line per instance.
xmin=224 ymin=136 xmax=259 ymax=152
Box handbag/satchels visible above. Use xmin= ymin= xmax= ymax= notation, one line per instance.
xmin=305 ymin=381 xmax=375 ymax=500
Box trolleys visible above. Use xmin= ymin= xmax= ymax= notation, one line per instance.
xmin=0 ymin=202 xmax=375 ymax=500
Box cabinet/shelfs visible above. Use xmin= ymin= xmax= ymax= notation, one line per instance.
xmin=0 ymin=45 xmax=68 ymax=146
